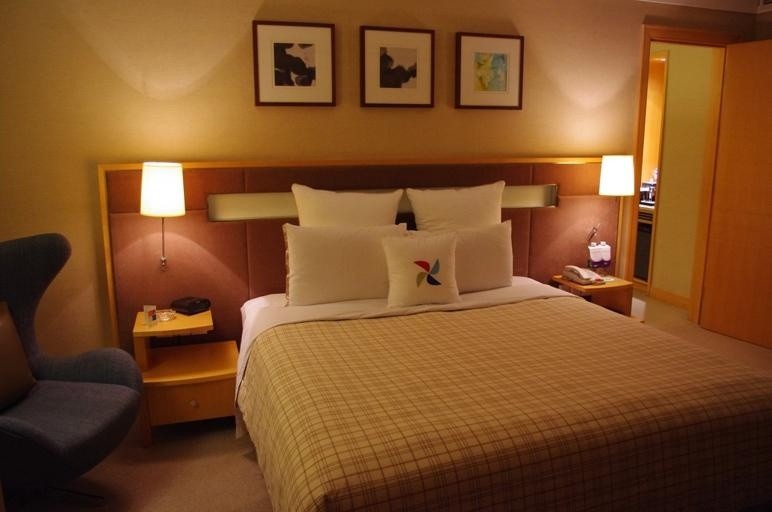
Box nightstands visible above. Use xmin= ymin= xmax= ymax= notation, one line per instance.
xmin=552 ymin=273 xmax=634 ymax=317
xmin=133 ymin=305 xmax=239 ymax=449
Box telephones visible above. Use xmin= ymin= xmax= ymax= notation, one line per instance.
xmin=562 ymin=265 xmax=606 ymax=285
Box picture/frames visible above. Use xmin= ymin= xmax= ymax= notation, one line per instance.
xmin=456 ymin=31 xmax=523 ymax=109
xmin=360 ymin=25 xmax=434 ymax=107
xmin=252 ymin=19 xmax=336 ymax=106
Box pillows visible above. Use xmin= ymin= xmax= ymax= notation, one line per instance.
xmin=406 ymin=180 xmax=505 ymax=233
xmin=291 ymin=183 xmax=404 ymax=227
xmin=382 ymin=232 xmax=461 ymax=306
xmin=409 ymin=219 xmax=513 ymax=294
xmin=282 ymin=222 xmax=406 ymax=307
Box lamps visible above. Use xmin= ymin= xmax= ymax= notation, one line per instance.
xmin=587 ymin=154 xmax=635 ymax=243
xmin=139 ymin=162 xmax=185 ymax=270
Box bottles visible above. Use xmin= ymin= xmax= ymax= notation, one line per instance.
xmin=585 ymin=240 xmax=612 ymax=278
xmin=648 ymin=187 xmax=655 ymax=201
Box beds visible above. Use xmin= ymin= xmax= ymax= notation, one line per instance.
xmin=234 ymin=274 xmax=772 ymax=511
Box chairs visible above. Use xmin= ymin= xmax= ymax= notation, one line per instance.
xmin=0 ymin=232 xmax=142 ymax=511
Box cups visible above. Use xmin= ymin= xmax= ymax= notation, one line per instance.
xmin=143 ymin=305 xmax=157 ymax=325
